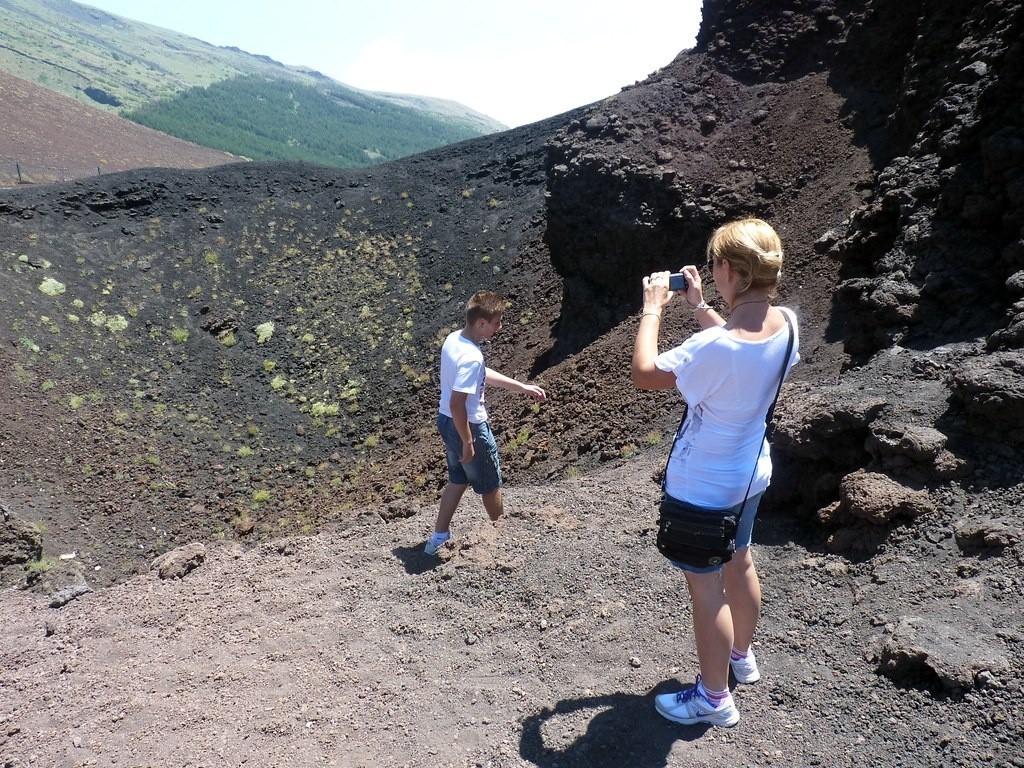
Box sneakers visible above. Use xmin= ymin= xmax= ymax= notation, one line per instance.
xmin=729 ymin=648 xmax=761 ymax=683
xmin=654 ymin=674 xmax=740 ymax=726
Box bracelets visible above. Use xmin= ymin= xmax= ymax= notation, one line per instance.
xmin=690 ymin=300 xmax=705 ymax=313
xmin=641 ymin=313 xmax=661 ymax=319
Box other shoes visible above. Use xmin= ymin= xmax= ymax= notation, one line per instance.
xmin=424 ymin=533 xmax=455 ymax=553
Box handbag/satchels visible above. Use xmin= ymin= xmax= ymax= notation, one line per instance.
xmin=655 ymin=500 xmax=738 ymax=567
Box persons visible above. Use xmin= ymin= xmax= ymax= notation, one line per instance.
xmin=424 ymin=290 xmax=546 ymax=555
xmin=630 ymin=219 xmax=801 ymax=729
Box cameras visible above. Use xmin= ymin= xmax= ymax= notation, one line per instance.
xmin=668 ymin=272 xmax=689 ymax=290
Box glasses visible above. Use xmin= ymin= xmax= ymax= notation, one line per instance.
xmin=707 ymin=258 xmax=720 ymax=272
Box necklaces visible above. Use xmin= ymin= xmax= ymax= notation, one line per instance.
xmin=730 ymin=298 xmax=768 ymax=313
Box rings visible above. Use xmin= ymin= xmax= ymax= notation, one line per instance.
xmin=651 ymin=278 xmax=655 ymax=281
xmin=656 ymin=276 xmax=663 ymax=280
